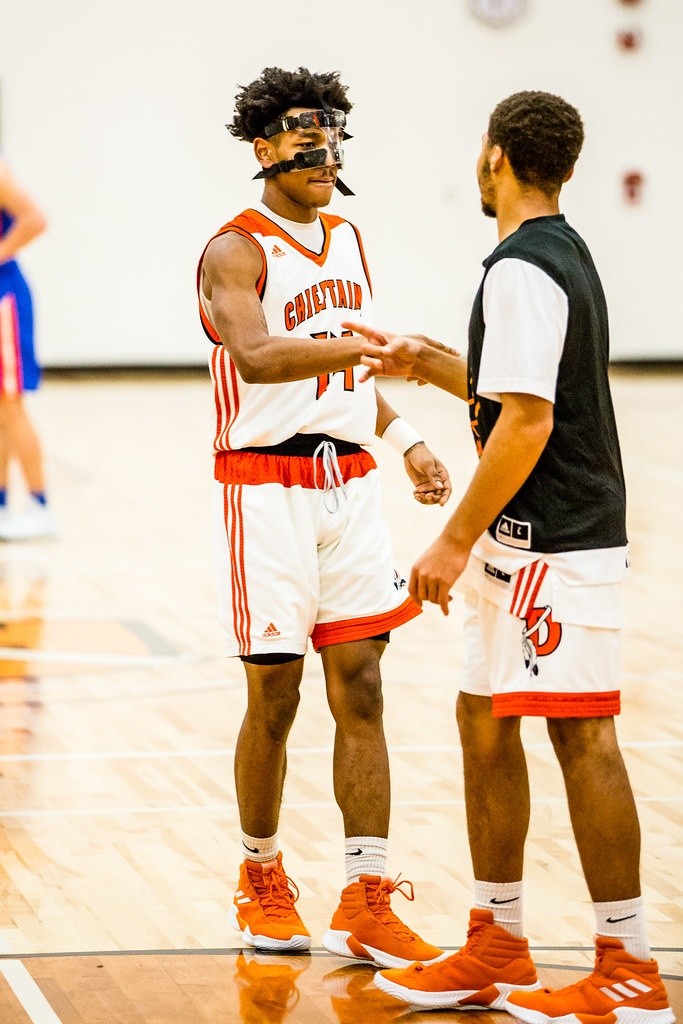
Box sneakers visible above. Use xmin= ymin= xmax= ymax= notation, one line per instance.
xmin=504 ymin=935 xmax=676 ymax=1024
xmin=228 ymin=851 xmax=311 ymax=951
xmin=373 ymin=908 xmax=542 ymax=1012
xmin=321 ymin=875 xmax=447 ymax=966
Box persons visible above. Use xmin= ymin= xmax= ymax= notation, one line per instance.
xmin=1 ymin=165 xmax=62 ymax=540
xmin=342 ymin=89 xmax=677 ymax=1024
xmin=195 ymin=62 xmax=462 ymax=970
